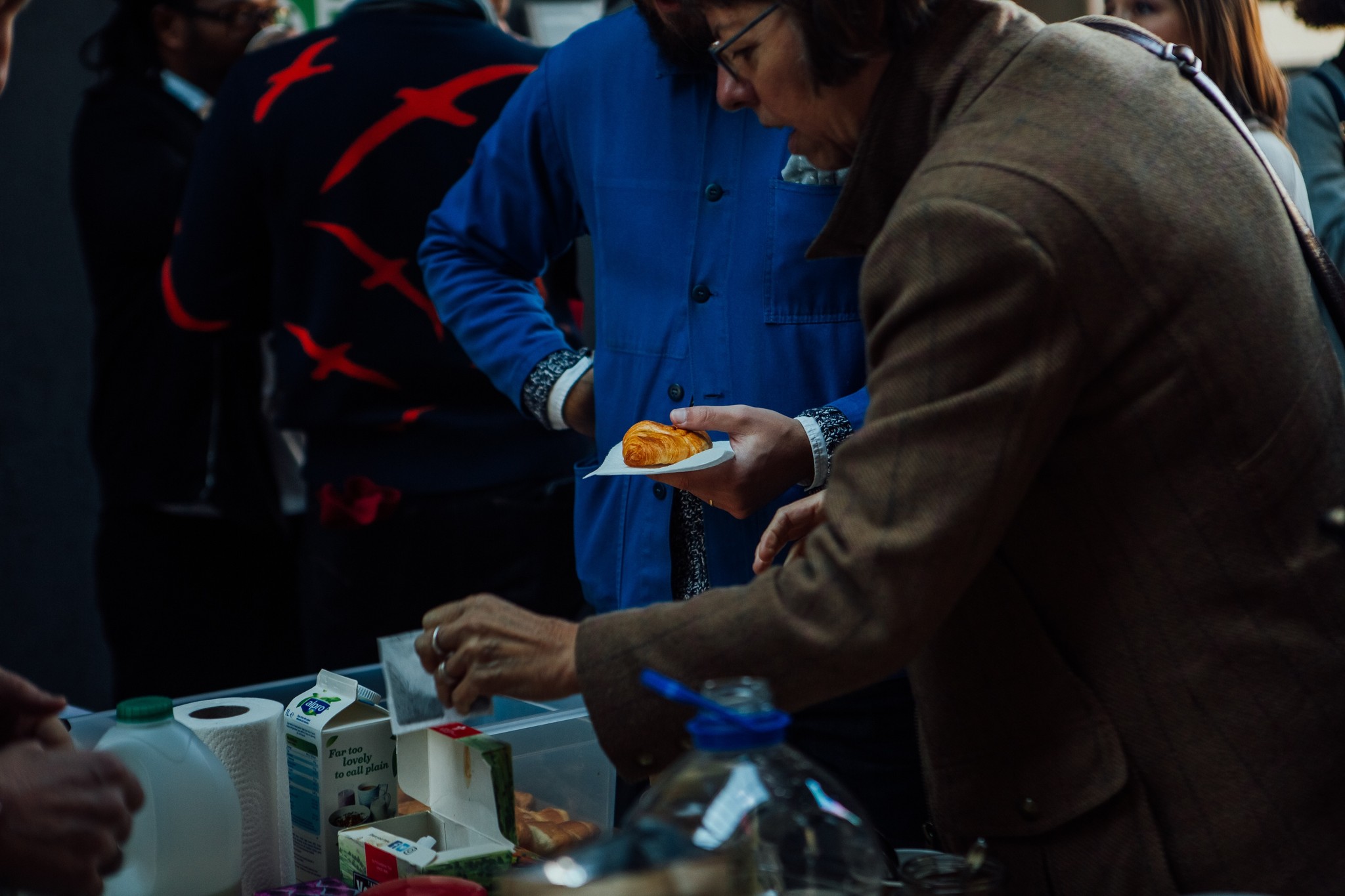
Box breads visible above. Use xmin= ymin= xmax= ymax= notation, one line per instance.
xmin=621 ymin=419 xmax=711 ymax=467
xmin=397 ymin=785 xmax=601 ymax=855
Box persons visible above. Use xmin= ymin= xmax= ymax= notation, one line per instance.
xmin=73 ymin=0 xmax=589 ymax=707
xmin=416 ymin=0 xmax=1345 ymax=896
xmin=0 ymin=0 xmax=147 ymax=896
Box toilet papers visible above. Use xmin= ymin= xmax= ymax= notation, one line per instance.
xmin=173 ymin=696 xmax=298 ymax=896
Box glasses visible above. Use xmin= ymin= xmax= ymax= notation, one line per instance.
xmin=172 ymin=2 xmax=277 ymax=39
xmin=707 ymin=4 xmax=800 ymax=92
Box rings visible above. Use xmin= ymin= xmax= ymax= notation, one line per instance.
xmin=437 ymin=660 xmax=459 ymax=688
xmin=432 ymin=625 xmax=455 ymax=657
xmin=709 ymin=499 xmax=713 ymax=507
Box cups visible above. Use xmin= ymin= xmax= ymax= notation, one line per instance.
xmin=849 ymin=848 xmax=945 ymax=896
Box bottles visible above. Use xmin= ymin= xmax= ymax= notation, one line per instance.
xmin=92 ymin=697 xmax=243 ymax=896
xmin=591 ymin=666 xmax=888 ymax=896
